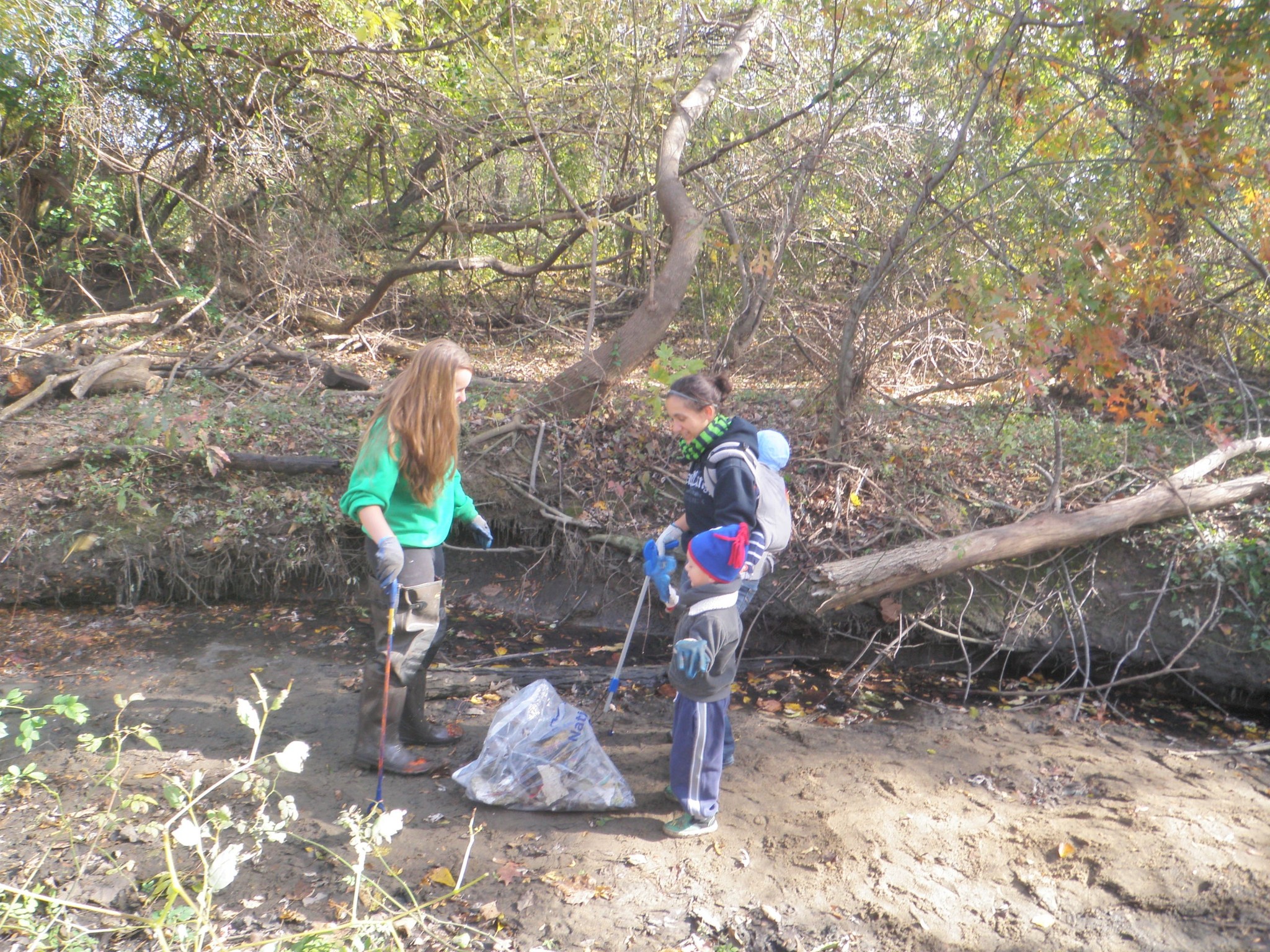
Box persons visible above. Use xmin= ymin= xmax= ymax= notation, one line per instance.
xmin=655 ymin=523 xmax=750 ymax=840
xmin=335 ymin=337 xmax=498 ymax=775
xmin=640 ymin=368 xmax=765 ymax=771
xmin=738 ymin=426 xmax=792 ymax=576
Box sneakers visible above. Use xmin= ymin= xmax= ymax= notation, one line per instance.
xmin=662 ymin=813 xmax=719 ymax=838
xmin=664 ymin=784 xmax=681 ymax=802
xmin=722 ymin=753 xmax=735 ymax=769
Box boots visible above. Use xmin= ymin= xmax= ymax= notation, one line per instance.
xmin=352 ymin=679 xmax=433 ymax=775
xmin=398 ymin=669 xmax=464 ymax=747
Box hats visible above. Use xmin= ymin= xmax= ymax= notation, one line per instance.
xmin=688 ymin=522 xmax=750 ymax=584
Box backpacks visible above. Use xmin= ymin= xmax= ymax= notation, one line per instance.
xmin=704 ymin=441 xmax=792 ymax=553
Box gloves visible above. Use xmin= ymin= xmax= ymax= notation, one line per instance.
xmin=654 ymin=521 xmax=685 ymax=558
xmin=653 ymin=575 xmax=680 ymax=611
xmin=374 ymin=536 xmax=405 ymax=590
xmin=674 ymin=638 xmax=712 ymax=679
xmin=467 ymin=514 xmax=493 ymax=550
